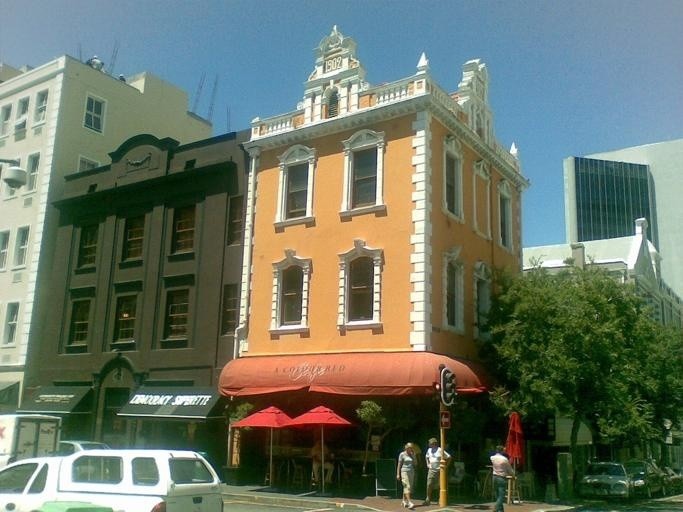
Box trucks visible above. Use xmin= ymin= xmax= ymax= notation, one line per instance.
xmin=0 ymin=413 xmax=225 ymax=512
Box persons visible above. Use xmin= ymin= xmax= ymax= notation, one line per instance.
xmin=90 ymin=56 xmax=102 ymax=72
xmin=310 ymin=438 xmax=334 ymax=485
xmin=119 ymin=74 xmax=125 ymax=82
xmin=489 ymin=445 xmax=516 ymax=511
xmin=422 ymin=437 xmax=452 ymax=504
xmin=395 ymin=442 xmax=419 ymax=510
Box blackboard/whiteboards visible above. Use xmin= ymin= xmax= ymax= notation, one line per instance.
xmin=376 ymin=458 xmax=397 ymax=490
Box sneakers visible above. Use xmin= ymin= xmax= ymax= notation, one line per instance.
xmin=402 ymin=500 xmax=414 ymax=508
xmin=423 ymin=499 xmax=430 ymax=506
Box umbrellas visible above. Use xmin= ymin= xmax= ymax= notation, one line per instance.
xmin=504 ymin=411 xmax=526 ymax=502
xmin=286 ymin=405 xmax=350 ymax=493
xmin=229 ymin=406 xmax=294 ymax=487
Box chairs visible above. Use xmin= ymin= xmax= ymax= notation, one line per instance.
xmin=258 ymin=450 xmax=535 ymax=506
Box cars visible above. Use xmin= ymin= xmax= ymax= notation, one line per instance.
xmin=574 ymin=458 xmax=683 ymax=505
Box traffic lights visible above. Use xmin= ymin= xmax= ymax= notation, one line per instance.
xmin=433 ymin=367 xmax=456 ymax=406
